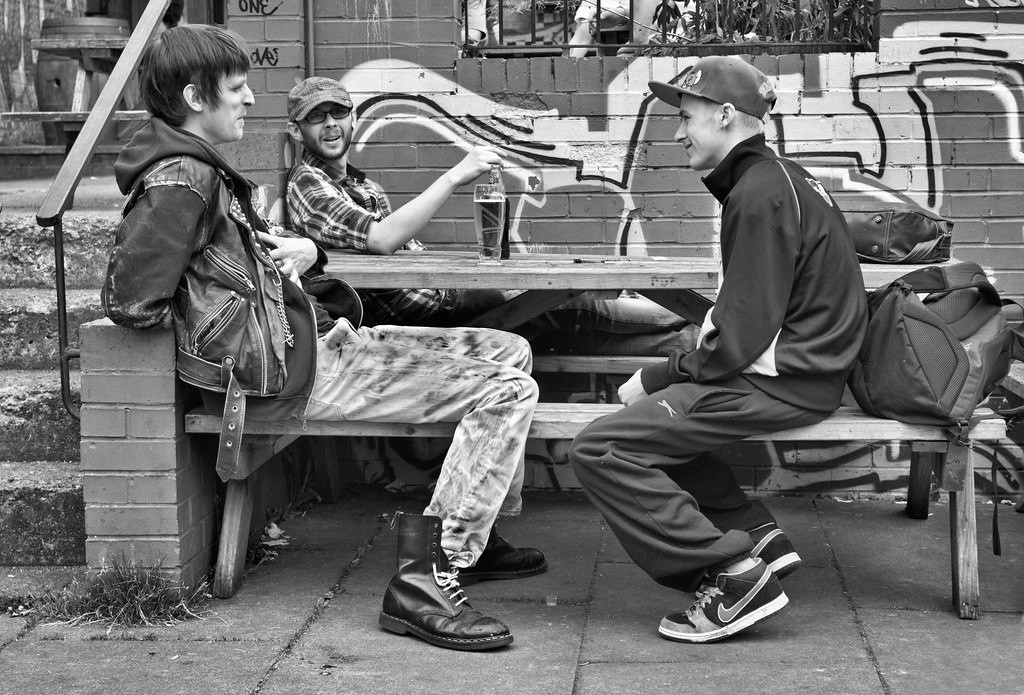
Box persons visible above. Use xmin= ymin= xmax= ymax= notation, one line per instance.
xmin=568 ymin=55 xmax=869 ymax=643
xmin=101 ymin=25 xmax=549 ymax=652
xmin=286 ymin=77 xmax=700 ymax=355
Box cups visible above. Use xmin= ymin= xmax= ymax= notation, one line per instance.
xmin=473 ymin=183 xmax=506 ymax=266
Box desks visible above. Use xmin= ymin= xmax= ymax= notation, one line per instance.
xmin=33 ymin=38 xmax=158 ymax=106
xmin=298 ymin=238 xmax=1000 ymax=355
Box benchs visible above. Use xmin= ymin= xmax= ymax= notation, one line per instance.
xmin=182 ymin=350 xmax=1024 ymax=617
xmin=0 ymin=109 xmax=156 ymax=156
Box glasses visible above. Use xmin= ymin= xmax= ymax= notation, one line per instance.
xmin=304 ymin=107 xmax=350 ymax=124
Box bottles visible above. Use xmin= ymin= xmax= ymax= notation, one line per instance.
xmin=482 ymin=164 xmax=510 ymax=260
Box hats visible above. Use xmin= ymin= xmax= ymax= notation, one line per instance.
xmin=287 ymin=77 xmax=353 ymax=121
xmin=648 ymin=56 xmax=778 ymax=125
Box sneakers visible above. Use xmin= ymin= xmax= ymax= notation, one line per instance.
xmin=747 ymin=521 xmax=801 ymax=579
xmin=658 ymin=558 xmax=790 ymax=644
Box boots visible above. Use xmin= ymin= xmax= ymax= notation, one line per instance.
xmin=379 ymin=511 xmax=514 ymax=650
xmin=449 ymin=524 xmax=548 ymax=587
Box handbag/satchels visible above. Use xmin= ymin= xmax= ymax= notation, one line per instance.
xmin=846 ymin=262 xmax=1024 ymax=444
xmin=835 ymin=200 xmax=953 ymax=263
